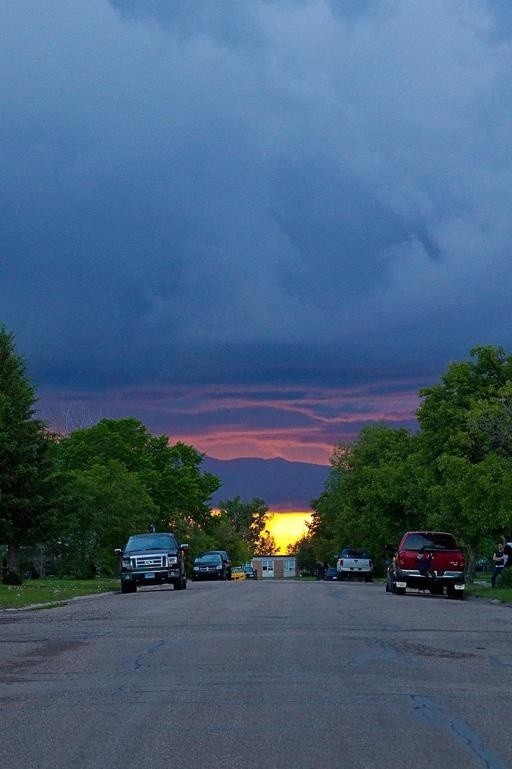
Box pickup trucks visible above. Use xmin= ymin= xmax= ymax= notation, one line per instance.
xmin=392 ymin=531 xmax=466 ymax=600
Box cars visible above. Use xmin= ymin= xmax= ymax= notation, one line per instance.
xmin=387 ymin=555 xmax=396 ymax=593
xmin=317 ymin=547 xmax=374 ymax=584
xmin=193 ymin=551 xmax=258 ymax=583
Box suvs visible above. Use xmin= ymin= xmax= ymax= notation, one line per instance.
xmin=113 ymin=533 xmax=189 ymax=592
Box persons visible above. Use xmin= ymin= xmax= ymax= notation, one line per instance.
xmin=490 ymin=542 xmax=504 ymax=585
xmin=502 ymin=536 xmax=512 ymax=568
